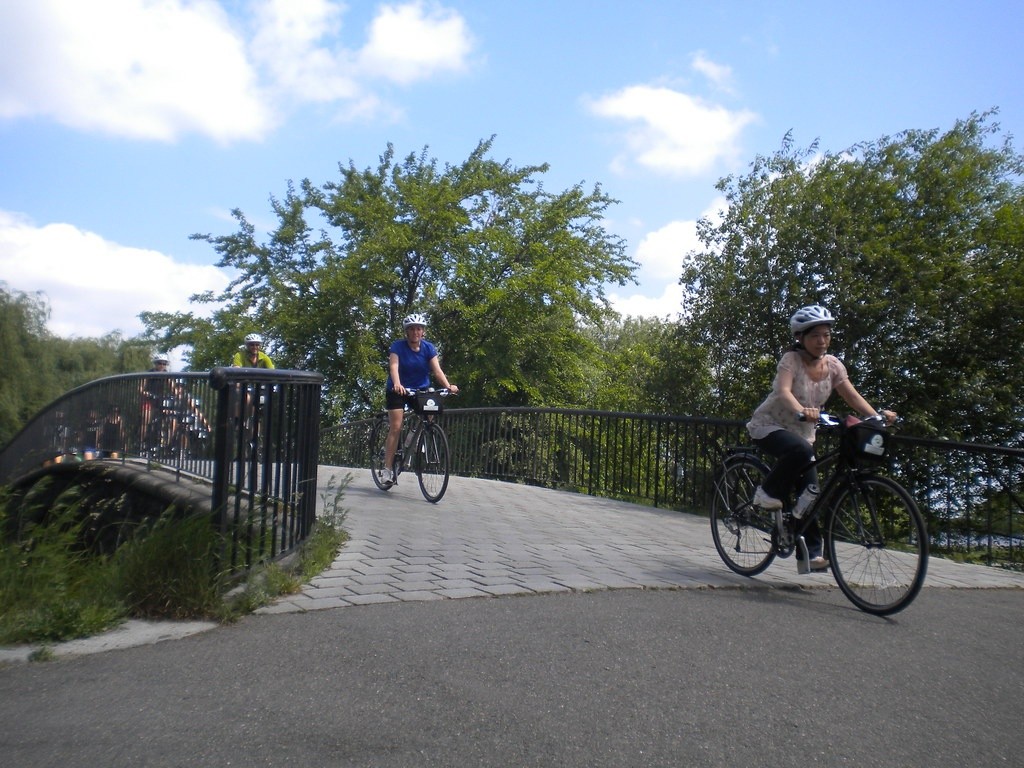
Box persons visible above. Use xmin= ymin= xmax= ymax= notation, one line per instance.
xmin=745 ymin=303 xmax=899 ymax=573
xmin=79 ymin=409 xmax=101 ymax=461
xmin=229 ymin=334 xmax=276 ymax=444
xmin=381 ymin=313 xmax=458 ymax=485
xmin=161 ymin=377 xmax=211 ymax=462
xmin=136 ymin=354 xmax=178 ymax=458
xmin=40 ymin=409 xmax=79 ymax=467
xmin=96 ymin=404 xmax=124 ymax=461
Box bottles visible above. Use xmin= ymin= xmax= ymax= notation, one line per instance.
xmin=404 ymin=427 xmax=418 ymax=448
xmin=792 ymin=483 xmax=819 ymax=519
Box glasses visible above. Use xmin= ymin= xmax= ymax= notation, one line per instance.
xmin=155 ymin=361 xmax=168 ymax=366
xmin=248 ymin=343 xmax=260 ymax=348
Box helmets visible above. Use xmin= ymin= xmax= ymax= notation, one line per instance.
xmin=243 ymin=333 xmax=263 ymax=345
xmin=401 ymin=313 xmax=427 ymax=329
xmin=790 ymin=305 xmax=836 ymax=335
xmin=152 ymin=354 xmax=168 ymax=363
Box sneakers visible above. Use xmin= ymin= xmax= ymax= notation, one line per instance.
xmin=379 ymin=464 xmax=393 ymax=485
xmin=751 ymin=484 xmax=783 ymax=511
xmin=795 ymin=553 xmax=832 ymax=576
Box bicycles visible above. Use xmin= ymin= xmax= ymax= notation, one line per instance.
xmin=367 ymin=384 xmax=453 ymax=506
xmin=709 ymin=409 xmax=927 ymax=618
